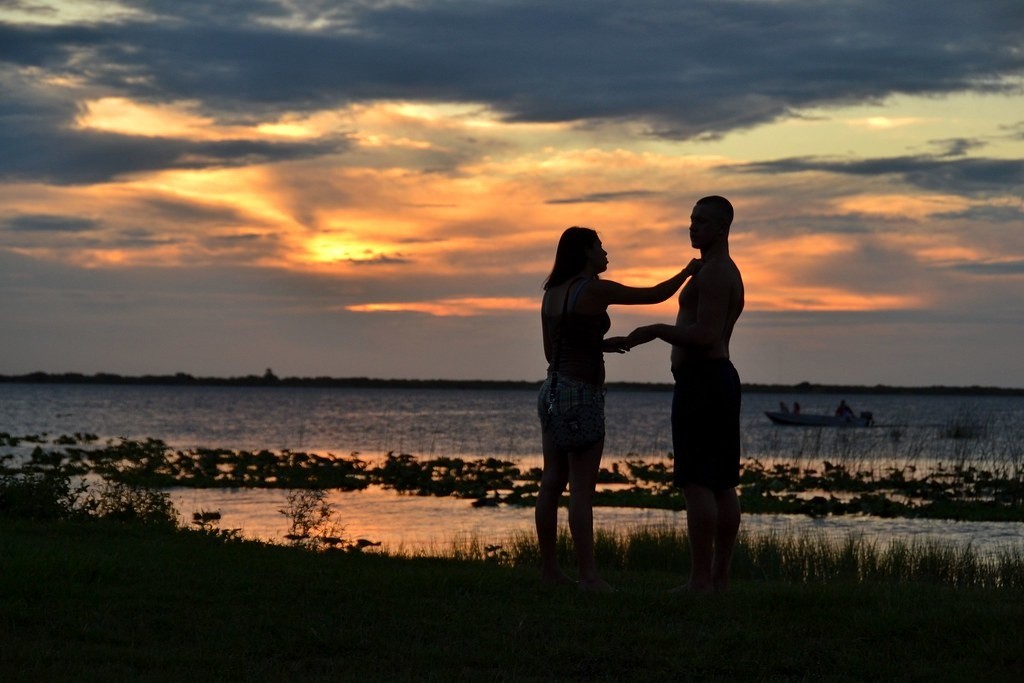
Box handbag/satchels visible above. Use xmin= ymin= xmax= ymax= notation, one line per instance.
xmin=549 ymin=403 xmax=605 ymax=451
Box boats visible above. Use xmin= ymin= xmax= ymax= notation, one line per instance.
xmin=764 ymin=409 xmax=873 ymax=428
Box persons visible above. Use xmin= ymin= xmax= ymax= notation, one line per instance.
xmin=779 ymin=400 xmax=855 ymax=418
xmin=534 ymin=227 xmax=702 ymax=591
xmin=628 ymin=196 xmax=744 ymax=591
